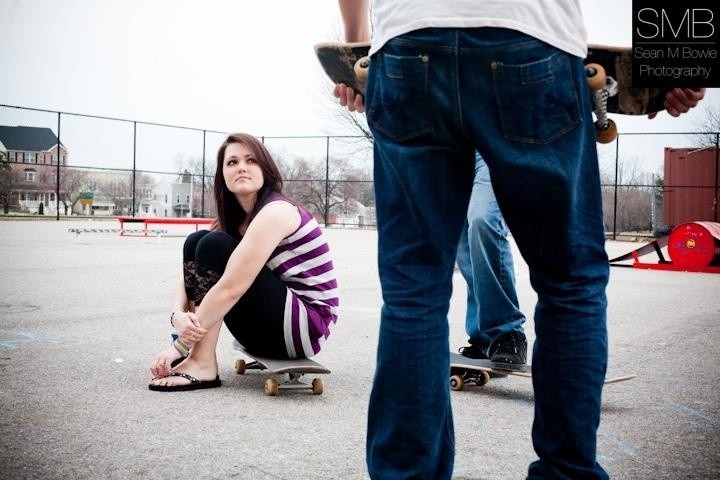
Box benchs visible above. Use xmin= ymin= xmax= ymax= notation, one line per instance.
xmin=119 ymin=217 xmax=217 ymax=236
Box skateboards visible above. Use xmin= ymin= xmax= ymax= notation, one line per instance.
xmin=313 ymin=41 xmax=698 ymax=143
xmin=449 ymin=351 xmax=637 ymax=392
xmin=232 ymin=339 xmax=331 ymax=396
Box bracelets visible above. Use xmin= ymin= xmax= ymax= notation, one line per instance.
xmin=171 ymin=337 xmax=192 ymax=360
xmin=170 ymin=312 xmax=176 ymax=328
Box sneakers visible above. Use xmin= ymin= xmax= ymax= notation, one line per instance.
xmin=457 ymin=330 xmax=528 ymax=379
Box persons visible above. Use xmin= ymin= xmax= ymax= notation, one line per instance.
xmin=330 ymin=0 xmax=709 ymax=480
xmin=450 ymin=148 xmax=530 ymax=376
xmin=149 ymin=132 xmax=341 ymax=393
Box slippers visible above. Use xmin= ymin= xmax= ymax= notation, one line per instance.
xmin=148 ymin=371 xmax=223 ymax=391
